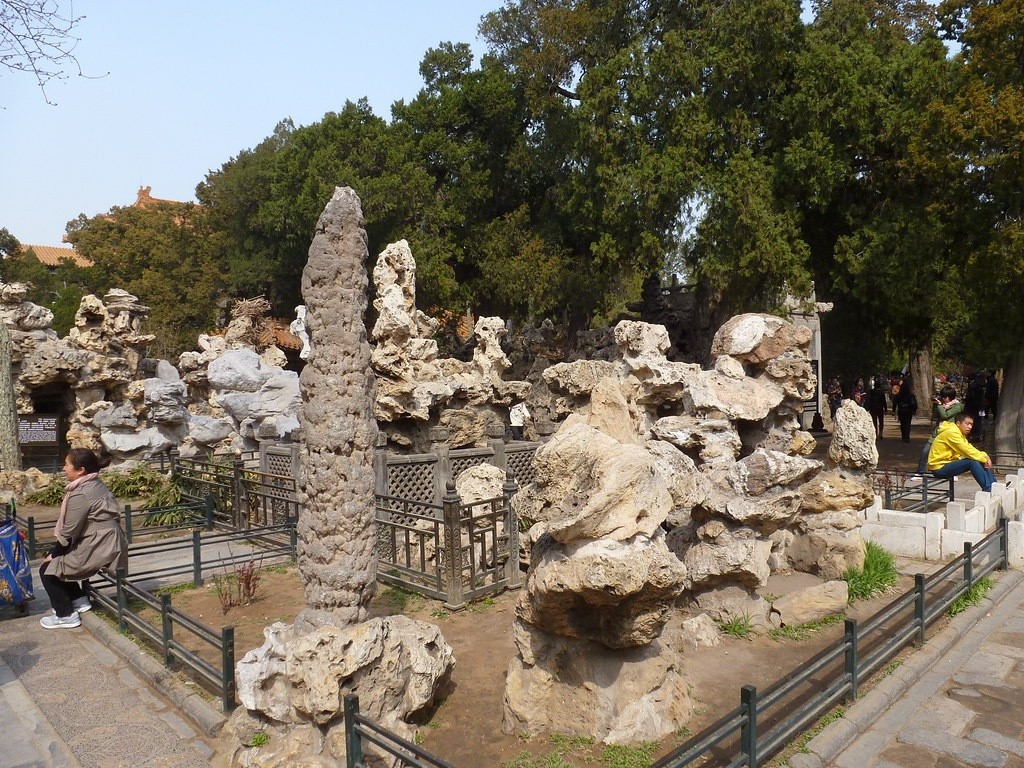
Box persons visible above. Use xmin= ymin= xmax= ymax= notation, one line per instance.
xmin=927 ymin=413 xmax=1011 ymax=493
xmin=39 ymin=448 xmax=127 ymax=628
xmin=825 ymin=368 xmax=999 ymax=442
xmin=909 ymin=386 xmax=959 ymax=482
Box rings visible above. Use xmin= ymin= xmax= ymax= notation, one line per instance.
xmin=987 ymin=462 xmax=989 ymax=464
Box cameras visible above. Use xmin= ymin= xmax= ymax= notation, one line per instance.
xmin=930 ymin=393 xmax=943 ymax=402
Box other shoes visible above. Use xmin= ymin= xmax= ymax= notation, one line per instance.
xmin=1006 ymin=481 xmax=1012 ymax=488
xmin=954 ymin=475 xmax=959 ymax=482
xmin=910 ymin=474 xmax=922 ymax=481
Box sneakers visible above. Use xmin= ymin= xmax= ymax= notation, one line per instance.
xmin=40 ymin=611 xmax=81 ymax=629
xmin=52 ymin=596 xmax=92 ymax=613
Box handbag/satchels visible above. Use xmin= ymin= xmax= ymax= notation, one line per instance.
xmin=909 ymin=395 xmax=917 ymax=412
